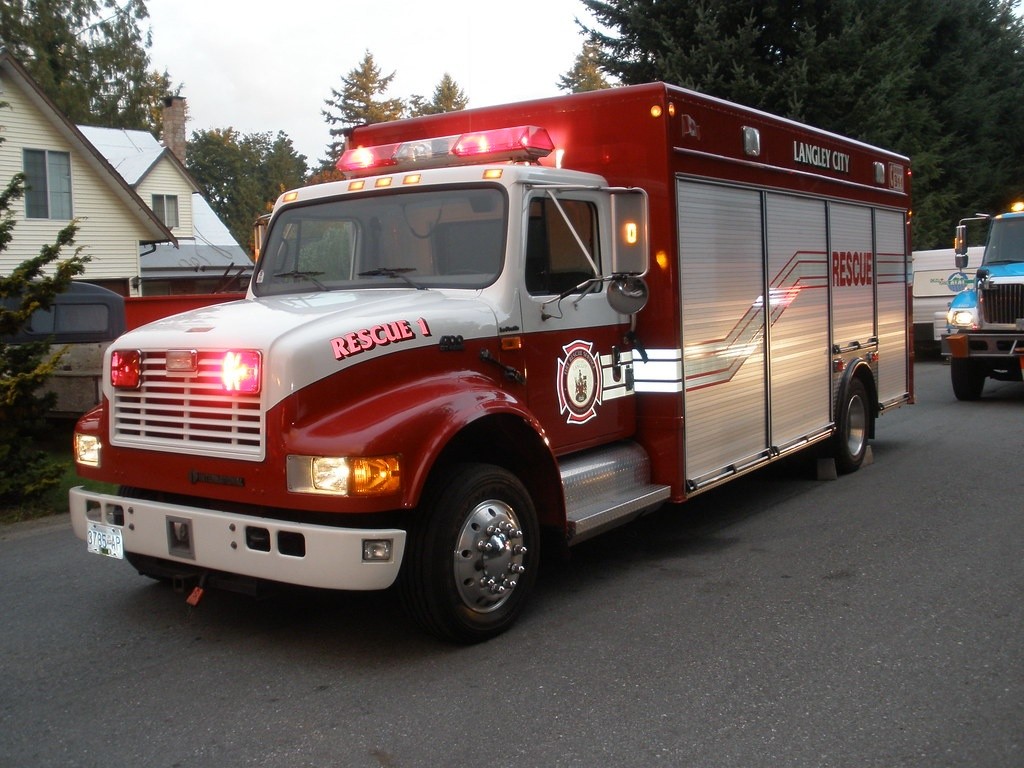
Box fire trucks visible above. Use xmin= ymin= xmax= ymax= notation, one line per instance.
xmin=65 ymin=75 xmax=915 ymax=647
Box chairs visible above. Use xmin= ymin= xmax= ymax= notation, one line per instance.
xmin=526 ymin=271 xmax=594 ymax=295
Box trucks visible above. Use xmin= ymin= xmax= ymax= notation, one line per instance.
xmin=936 ymin=203 xmax=1024 ymax=401
